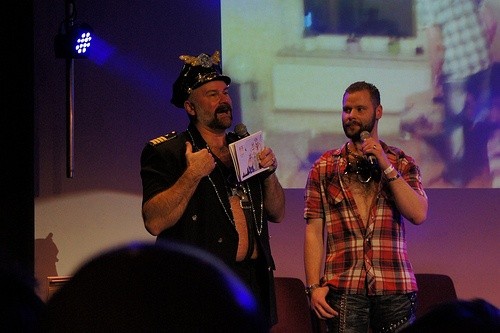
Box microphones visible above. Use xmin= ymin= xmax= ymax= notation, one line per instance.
xmin=359 ymin=131 xmax=377 ymax=165
xmin=235 ymin=123 xmax=276 ymax=174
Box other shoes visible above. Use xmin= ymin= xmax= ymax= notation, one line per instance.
xmin=441 ymin=169 xmax=466 ymax=187
xmin=474 ymin=167 xmax=480 ymax=174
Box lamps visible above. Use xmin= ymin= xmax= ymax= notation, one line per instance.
xmin=55 ymin=17 xmax=96 ymax=59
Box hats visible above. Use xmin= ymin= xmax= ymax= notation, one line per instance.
xmin=171 ymin=54 xmax=231 ymax=108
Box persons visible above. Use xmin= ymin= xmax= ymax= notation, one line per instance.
xmin=303 ymin=79 xmax=429 ymax=333
xmin=47 ymin=241 xmax=271 ymax=333
xmin=398 ymin=297 xmax=500 ymax=333
xmin=140 ymin=51 xmax=286 ymax=328
xmin=415 ymin=0 xmax=496 ymax=187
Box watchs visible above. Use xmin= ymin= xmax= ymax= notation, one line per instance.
xmin=305 ymin=283 xmax=318 ymax=294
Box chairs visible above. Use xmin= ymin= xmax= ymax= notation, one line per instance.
xmin=414 ymin=274 xmax=458 ymax=318
xmin=270 ymin=277 xmax=328 ymax=333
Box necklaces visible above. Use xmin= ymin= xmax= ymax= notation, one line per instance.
xmin=188 ymin=130 xmax=265 ymax=236
xmin=347 ymin=145 xmax=377 ymax=183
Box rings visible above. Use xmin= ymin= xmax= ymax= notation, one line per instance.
xmin=374 ymin=145 xmax=376 ymax=149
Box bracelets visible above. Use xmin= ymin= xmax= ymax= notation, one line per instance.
xmin=384 ymin=164 xmax=395 ymax=174
xmin=389 ymin=172 xmax=400 ymax=181
